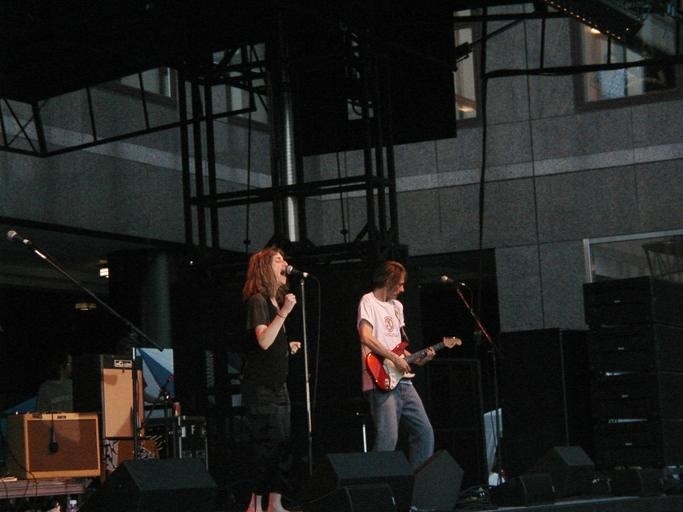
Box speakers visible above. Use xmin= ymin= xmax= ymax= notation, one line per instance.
xmin=526 ymin=445 xmax=594 ymax=500
xmin=77 ymin=458 xmax=219 ymax=512
xmin=582 ymin=274 xmax=683 ymax=470
xmin=426 ymin=357 xmax=482 ymax=485
xmin=304 ymin=450 xmax=415 ymax=512
xmin=103 ymin=439 xmax=161 ymax=479
xmin=5 ymin=411 xmax=101 ymax=481
xmin=492 ymin=326 xmax=616 ymax=497
xmin=78 ymin=353 xmax=146 ymax=439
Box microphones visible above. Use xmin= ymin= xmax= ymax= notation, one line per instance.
xmin=287 ymin=264 xmax=312 ymax=279
xmin=49 ymin=424 xmax=58 ymax=453
xmin=7 ymin=229 xmax=33 ymax=247
xmin=440 ymin=275 xmax=466 ymax=286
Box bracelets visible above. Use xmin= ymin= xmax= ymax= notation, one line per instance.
xmin=277 ymin=313 xmax=286 ymax=319
xmin=651 ymin=77 xmax=657 ymax=84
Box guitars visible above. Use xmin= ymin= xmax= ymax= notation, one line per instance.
xmin=365 ymin=335 xmax=462 ymax=391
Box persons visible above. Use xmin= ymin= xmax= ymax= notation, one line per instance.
xmin=590 ymin=52 xmax=639 ymax=102
xmin=241 ymin=245 xmax=303 ymax=511
xmin=635 ymin=46 xmax=670 ymax=94
xmin=355 ymin=261 xmax=435 ymax=474
